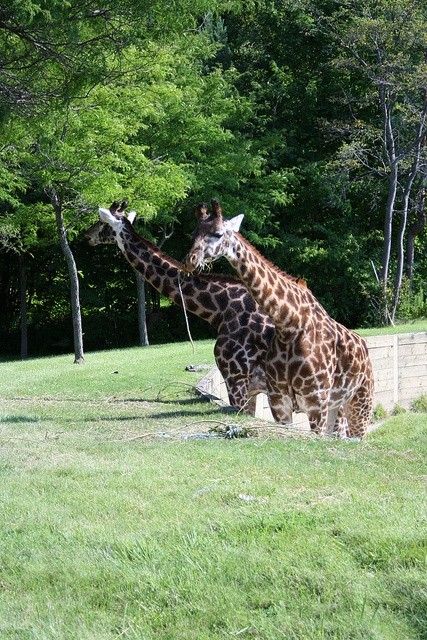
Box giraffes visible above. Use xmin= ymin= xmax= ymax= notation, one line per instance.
xmin=84 ymin=200 xmax=348 ymax=437
xmin=181 ymin=199 xmax=373 ymax=440
